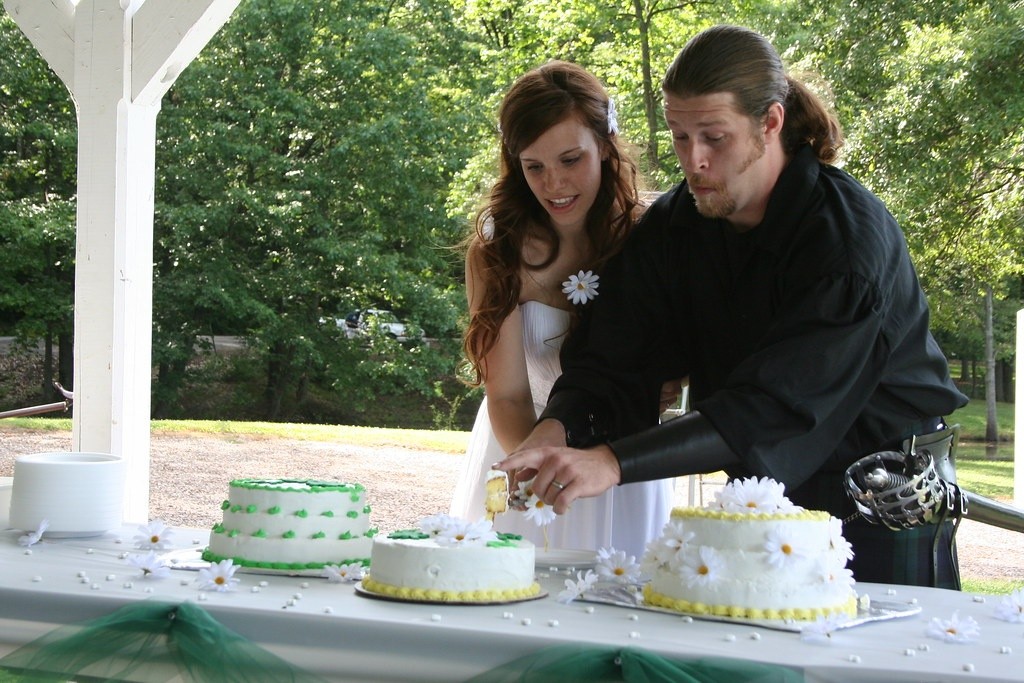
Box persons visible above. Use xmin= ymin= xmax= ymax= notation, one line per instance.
xmin=459 ymin=62 xmax=701 ymax=568
xmin=489 ymin=25 xmax=972 ymax=590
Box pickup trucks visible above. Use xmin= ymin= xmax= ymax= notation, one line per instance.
xmin=319 ymin=310 xmax=425 ymax=345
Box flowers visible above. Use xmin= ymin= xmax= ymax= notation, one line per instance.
xmin=926 ymin=610 xmax=980 ymax=644
xmin=420 ymin=512 xmax=497 ymax=547
xmin=991 ymin=587 xmax=1024 ymax=623
xmin=18 ymin=517 xmax=51 ymax=548
xmin=515 ymin=477 xmax=558 ymax=527
xmin=320 ymin=560 xmax=366 ymax=585
xmin=195 ymin=558 xmax=243 ymax=591
xmin=606 ymin=96 xmax=619 ymax=135
xmin=133 ymin=518 xmax=176 ymax=550
xmin=560 ymin=475 xmax=858 ymax=640
xmin=124 ymin=549 xmax=170 ymax=578
xmin=561 ymin=270 xmax=599 ymax=304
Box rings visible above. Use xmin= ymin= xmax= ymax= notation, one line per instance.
xmin=552 ymin=481 xmax=563 ymax=490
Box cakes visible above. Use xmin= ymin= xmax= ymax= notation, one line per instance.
xmin=200 ymin=477 xmax=374 ymax=568
xmin=362 ymin=467 xmax=540 ymax=602
xmin=643 ymin=474 xmax=861 ymax=622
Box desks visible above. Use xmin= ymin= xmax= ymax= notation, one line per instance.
xmin=0 ymin=521 xmax=1024 ymax=683
